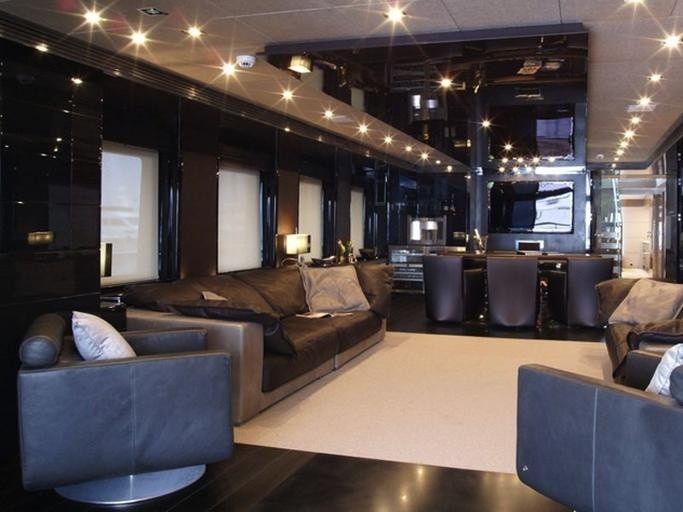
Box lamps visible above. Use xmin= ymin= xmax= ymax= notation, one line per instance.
xmin=288 ymin=56 xmax=314 ymax=75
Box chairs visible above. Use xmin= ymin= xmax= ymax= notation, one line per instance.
xmin=422 ymin=252 xmax=614 ymax=331
xmin=16 ymin=310 xmax=235 ymax=508
xmin=515 ymin=348 xmax=683 ymax=512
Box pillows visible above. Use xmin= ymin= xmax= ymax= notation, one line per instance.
xmin=70 ymin=309 xmax=135 ymax=362
xmin=608 ymin=278 xmax=683 ymax=327
xmin=298 ymin=263 xmax=371 ymax=316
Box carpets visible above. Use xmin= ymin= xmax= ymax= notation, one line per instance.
xmin=229 ymin=328 xmax=620 ymax=477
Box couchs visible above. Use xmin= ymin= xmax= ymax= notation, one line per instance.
xmin=594 ymin=275 xmax=683 ymax=378
xmin=123 ymin=259 xmax=393 ymax=427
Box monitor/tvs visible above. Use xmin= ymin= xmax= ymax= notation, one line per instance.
xmin=488 ymin=103 xmax=574 ymax=160
xmin=488 ymin=180 xmax=575 ymax=234
